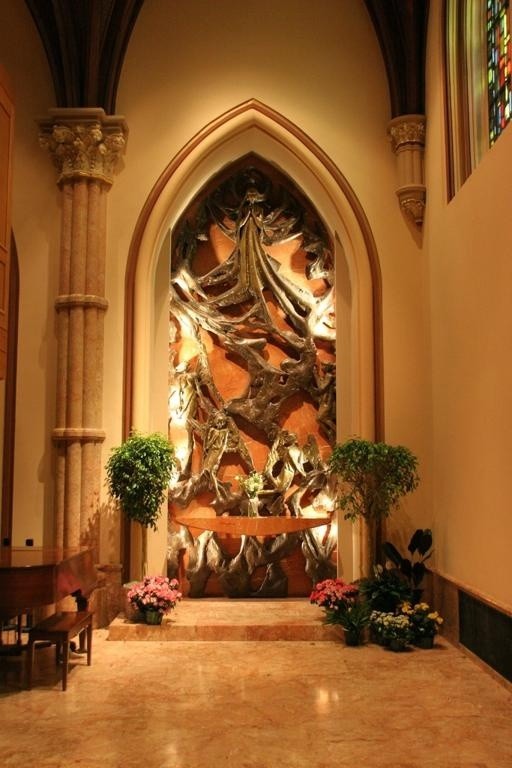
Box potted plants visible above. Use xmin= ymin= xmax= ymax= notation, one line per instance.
xmin=104 ymin=426 xmax=168 ymax=622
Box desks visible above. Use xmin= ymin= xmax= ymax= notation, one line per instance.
xmin=0 ymin=545 xmax=95 ymax=653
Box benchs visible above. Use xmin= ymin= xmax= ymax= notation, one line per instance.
xmin=25 ymin=611 xmax=94 ymax=690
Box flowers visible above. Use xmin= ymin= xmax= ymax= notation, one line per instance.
xmin=311 ymin=565 xmax=442 ymax=652
xmin=236 ymin=473 xmax=267 ymax=516
xmin=125 ymin=575 xmax=183 ymax=613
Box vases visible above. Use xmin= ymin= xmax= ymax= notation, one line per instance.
xmin=247 ymin=498 xmax=259 ymax=517
xmin=145 ymin=609 xmax=163 ymax=625
